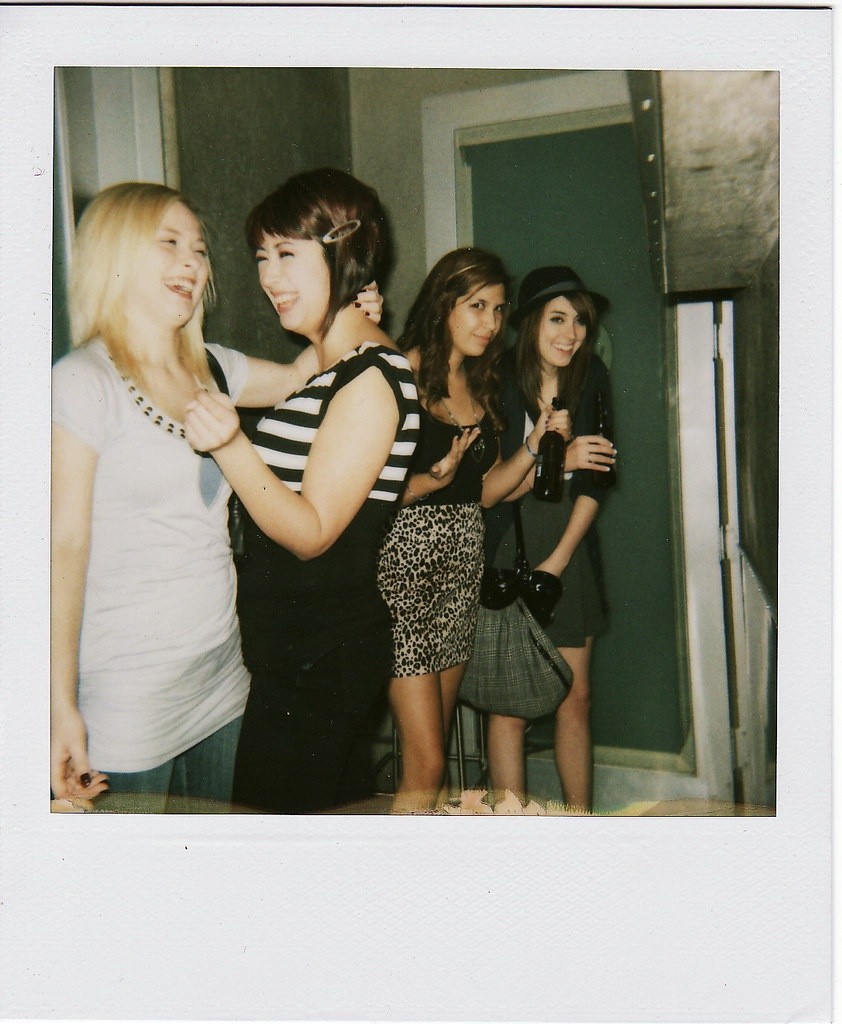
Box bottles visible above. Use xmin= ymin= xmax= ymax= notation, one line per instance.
xmin=534 ymin=396 xmax=567 ymax=506
xmin=590 ymin=390 xmax=619 ymax=489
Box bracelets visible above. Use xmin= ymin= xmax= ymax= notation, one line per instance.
xmin=524 ymin=435 xmax=539 ymax=460
xmin=406 ymin=472 xmax=429 ymax=503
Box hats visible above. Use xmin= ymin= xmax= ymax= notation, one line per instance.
xmin=507 ymin=266 xmax=610 ymax=331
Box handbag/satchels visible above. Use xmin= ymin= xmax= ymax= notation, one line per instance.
xmin=457 ymin=597 xmax=574 ymax=718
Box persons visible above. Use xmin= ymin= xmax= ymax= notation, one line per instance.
xmin=469 ymin=260 xmax=619 ymax=816
xmin=184 ymin=165 xmax=419 ymax=816
xmin=50 ymin=181 xmax=387 ymax=815
xmin=374 ymin=245 xmax=574 ymax=816
